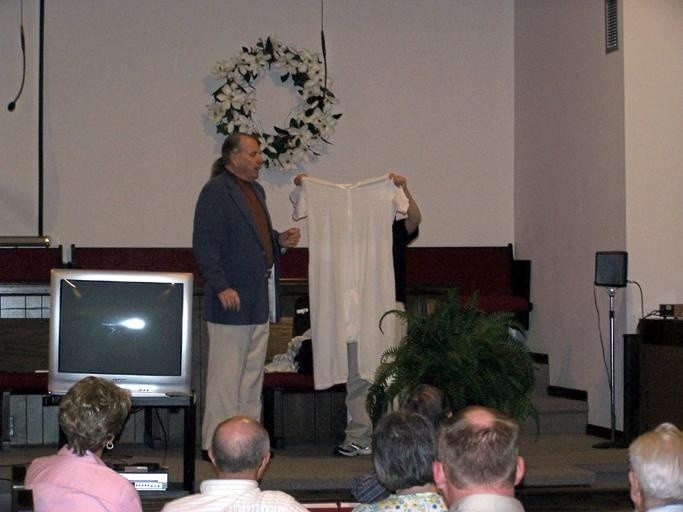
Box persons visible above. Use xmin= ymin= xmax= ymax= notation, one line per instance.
xmin=628 ymin=423 xmax=683 ymax=512
xmin=294 ymin=173 xmax=421 ymax=457
xmin=192 ymin=132 xmax=301 ymax=461
xmin=351 ymin=385 xmax=526 ymax=512
xmin=161 ymin=417 xmax=311 ymax=512
xmin=25 ymin=376 xmax=143 ymax=512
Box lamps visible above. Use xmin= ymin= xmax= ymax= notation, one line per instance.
xmin=592 ymin=251 xmax=630 ymax=449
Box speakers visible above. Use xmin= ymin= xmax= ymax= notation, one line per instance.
xmin=595 ymin=250 xmax=627 ymax=288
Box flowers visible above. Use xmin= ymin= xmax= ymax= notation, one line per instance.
xmin=209 ymin=37 xmax=338 ymax=169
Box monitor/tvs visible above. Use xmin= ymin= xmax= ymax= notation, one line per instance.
xmin=48 ymin=268 xmax=193 ymax=397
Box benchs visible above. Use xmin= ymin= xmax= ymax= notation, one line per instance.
xmin=1 ymin=317 xmax=156 ymax=450
xmin=70 ymin=243 xmax=533 ymax=332
xmin=262 ymin=372 xmax=347 ymax=450
xmin=0 ymin=244 xmax=66 ymax=296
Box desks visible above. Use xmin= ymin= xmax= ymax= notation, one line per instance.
xmin=41 ymin=392 xmax=197 ymax=494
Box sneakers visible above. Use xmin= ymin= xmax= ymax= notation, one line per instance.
xmin=333 ymin=443 xmax=373 ymax=457
